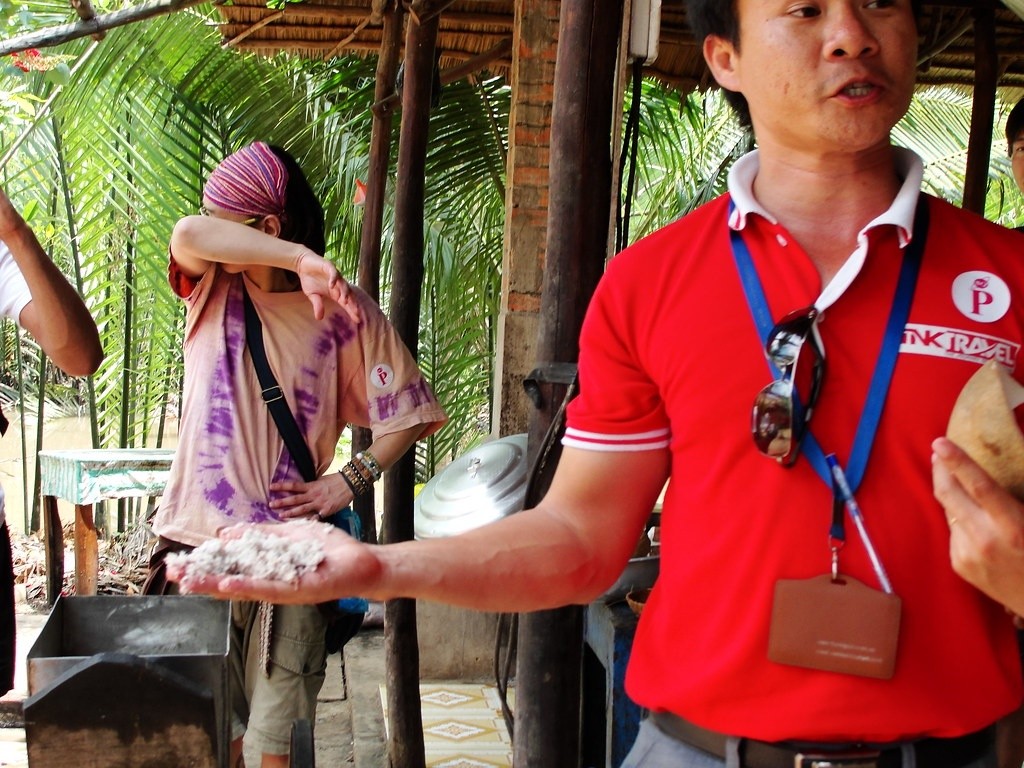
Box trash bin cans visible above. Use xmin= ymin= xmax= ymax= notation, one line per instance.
xmin=22 ymin=592 xmax=237 ymax=768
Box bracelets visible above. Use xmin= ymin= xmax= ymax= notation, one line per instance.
xmin=338 ymin=450 xmax=384 ymax=499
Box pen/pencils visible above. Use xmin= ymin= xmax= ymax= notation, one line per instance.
xmin=825 ymin=451 xmax=892 ymax=594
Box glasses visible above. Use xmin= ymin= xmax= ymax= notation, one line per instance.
xmin=750 ymin=304 xmax=827 ymax=467
xmin=199 ymin=206 xmax=258 ymax=232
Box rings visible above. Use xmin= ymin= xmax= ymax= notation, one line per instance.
xmin=317 ymin=511 xmax=325 ymax=521
xmin=946 ymin=517 xmax=960 ymax=531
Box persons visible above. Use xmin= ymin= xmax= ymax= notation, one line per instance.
xmin=160 ymin=0 xmax=1024 ymax=768
xmin=1003 ymin=95 xmax=1024 ymax=237
xmin=0 ymin=184 xmax=105 ymax=695
xmin=132 ymin=140 xmax=451 ymax=768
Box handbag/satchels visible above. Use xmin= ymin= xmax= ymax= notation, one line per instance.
xmin=320 ymin=506 xmax=368 ymax=654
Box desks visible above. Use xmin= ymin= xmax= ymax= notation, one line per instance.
xmin=37 ymin=449 xmax=174 ymax=606
xmin=582 ymin=600 xmax=643 ymax=768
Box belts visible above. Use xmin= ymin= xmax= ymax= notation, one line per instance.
xmin=650 ymin=712 xmax=996 ymax=768
